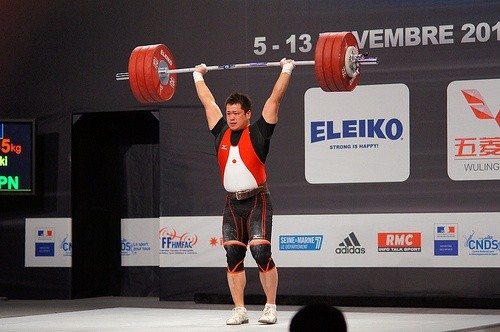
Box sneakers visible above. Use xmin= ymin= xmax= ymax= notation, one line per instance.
xmin=226 ymin=307 xmax=249 ymax=325
xmin=258 ymin=303 xmax=277 ymax=324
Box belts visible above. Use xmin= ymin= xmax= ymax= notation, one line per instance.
xmin=227 ymin=186 xmax=263 ymax=200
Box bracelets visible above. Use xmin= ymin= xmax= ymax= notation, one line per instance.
xmin=281 ymin=63 xmax=294 ymax=75
xmin=192 ymin=71 xmax=204 ymax=84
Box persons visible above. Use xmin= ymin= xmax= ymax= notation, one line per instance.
xmin=289 ymin=304 xmax=347 ymax=332
xmin=192 ymin=57 xmax=295 ymax=325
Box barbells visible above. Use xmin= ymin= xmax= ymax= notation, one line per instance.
xmin=114 ymin=32 xmax=380 ymax=105
